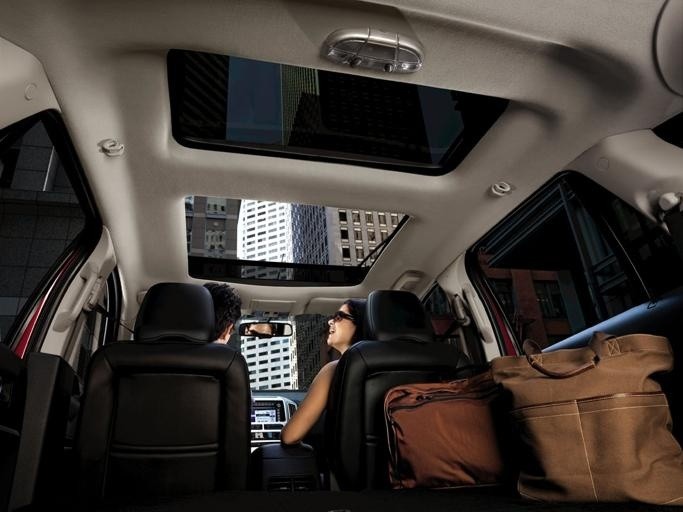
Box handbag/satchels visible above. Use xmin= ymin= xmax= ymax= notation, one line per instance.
xmin=487 ymin=328 xmax=682 ymax=508
xmin=381 ymin=370 xmax=525 ymax=493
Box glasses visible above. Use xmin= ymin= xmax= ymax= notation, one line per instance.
xmin=246 ymin=327 xmax=273 ymax=340
xmin=331 ymin=311 xmax=358 ymax=324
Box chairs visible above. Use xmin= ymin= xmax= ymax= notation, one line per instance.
xmin=70 ymin=281 xmax=252 ymax=512
xmin=318 ymin=289 xmax=459 ymax=496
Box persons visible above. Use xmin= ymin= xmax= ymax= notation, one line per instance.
xmin=203 ymin=279 xmax=243 ymax=345
xmin=279 ymin=299 xmax=368 ymax=446
xmin=244 ymin=323 xmax=274 ymax=336
